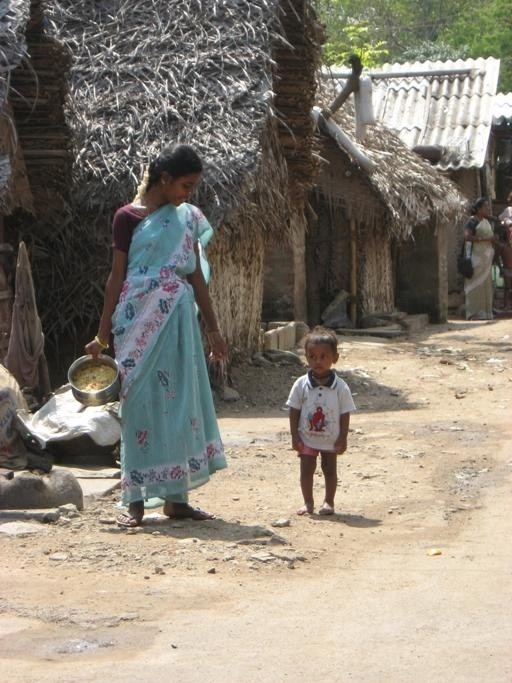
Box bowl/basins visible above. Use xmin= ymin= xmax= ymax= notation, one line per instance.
xmin=67 ymin=354 xmax=121 ymax=406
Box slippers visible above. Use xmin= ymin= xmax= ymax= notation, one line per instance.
xmin=169 ymin=506 xmax=216 ymax=520
xmin=115 ymin=511 xmax=143 ymax=527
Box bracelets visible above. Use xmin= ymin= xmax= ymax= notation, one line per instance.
xmin=205 ymin=328 xmax=220 ymax=333
xmin=94 ymin=333 xmax=110 ymax=350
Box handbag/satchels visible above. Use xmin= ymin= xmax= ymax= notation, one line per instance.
xmin=456 ymin=254 xmax=473 ymax=279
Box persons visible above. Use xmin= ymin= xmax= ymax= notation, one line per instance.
xmin=82 ymin=141 xmax=237 ymax=529
xmin=498 ymin=190 xmax=512 ymax=311
xmin=461 ymin=195 xmax=501 ymax=321
xmin=283 ymin=326 xmax=355 ymax=519
